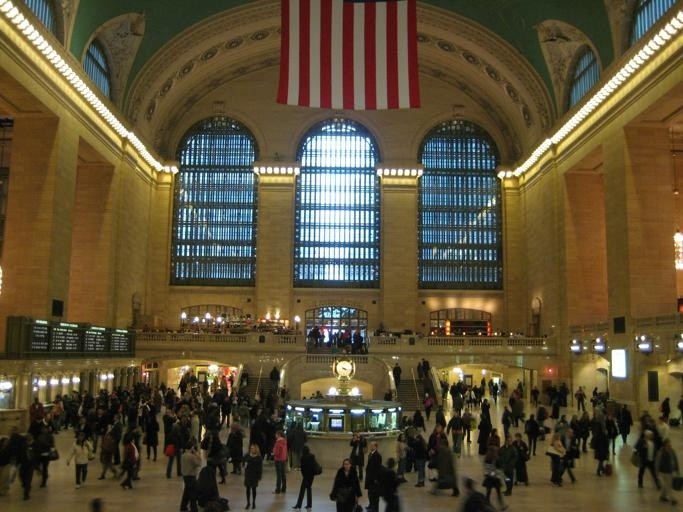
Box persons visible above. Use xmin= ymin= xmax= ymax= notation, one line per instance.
xmin=1 ymin=319 xmax=683 ymax=511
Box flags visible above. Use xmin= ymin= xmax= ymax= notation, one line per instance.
xmin=277 ymin=0 xmax=421 ymax=111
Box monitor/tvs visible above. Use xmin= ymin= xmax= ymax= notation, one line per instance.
xmin=330 ymin=417 xmax=344 ymax=431
xmin=309 ymin=422 xmax=320 ymax=431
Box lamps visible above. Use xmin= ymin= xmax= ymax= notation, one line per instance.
xmin=332 ymin=355 xmax=356 ymax=395
